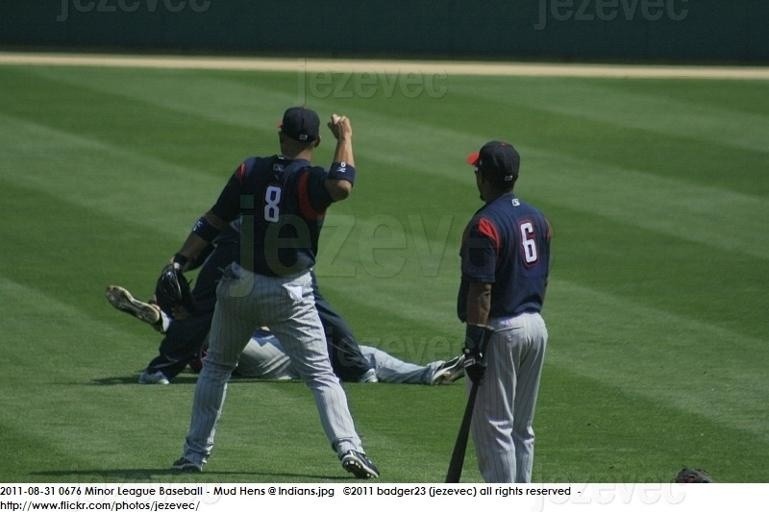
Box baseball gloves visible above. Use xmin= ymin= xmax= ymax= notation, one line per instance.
xmin=154 ymin=263 xmax=192 ymax=318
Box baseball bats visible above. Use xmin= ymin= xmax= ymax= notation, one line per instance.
xmin=443 ymin=324 xmax=500 ymax=484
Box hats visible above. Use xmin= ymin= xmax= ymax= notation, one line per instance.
xmin=278 ymin=106 xmax=320 ymax=139
xmin=466 ymin=140 xmax=520 ymax=176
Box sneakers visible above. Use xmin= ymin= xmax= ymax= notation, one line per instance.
xmin=104 ymin=284 xmax=160 ymax=324
xmin=431 ymin=354 xmax=467 ymax=386
xmin=173 ymin=453 xmax=203 ymax=473
xmin=358 ymin=368 xmax=380 ymax=383
xmin=137 ymin=370 xmax=171 ymax=385
xmin=340 ymin=450 xmax=380 ymax=480
